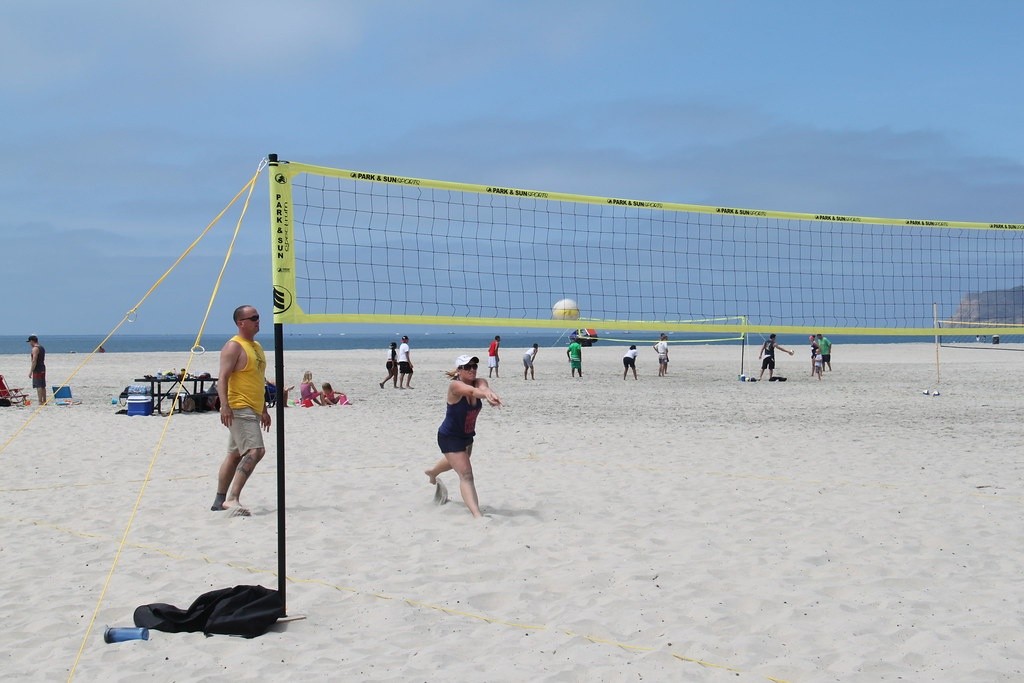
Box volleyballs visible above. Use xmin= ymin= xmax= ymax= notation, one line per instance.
xmin=552 ymin=296 xmax=582 ymax=322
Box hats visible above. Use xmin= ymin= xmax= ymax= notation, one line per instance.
xmin=26 ymin=335 xmax=38 ymax=342
xmin=455 ymin=355 xmax=480 ymax=368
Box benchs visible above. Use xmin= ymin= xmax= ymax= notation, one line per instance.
xmin=167 ymin=393 xmax=218 ymax=413
xmin=120 ymin=393 xmax=170 ymax=398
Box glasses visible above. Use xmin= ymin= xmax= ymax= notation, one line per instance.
xmin=459 ymin=364 xmax=478 ymax=371
xmin=240 ymin=315 xmax=260 ymax=321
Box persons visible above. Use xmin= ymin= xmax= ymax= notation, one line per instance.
xmin=99 ymin=346 xmax=105 ymax=353
xmin=488 ymin=335 xmax=501 ymax=378
xmin=654 ymin=333 xmax=669 ymax=377
xmin=301 ymin=371 xmax=353 ymax=406
xmin=379 ymin=335 xmax=414 ymax=390
xmin=567 ymin=335 xmax=582 ymax=377
xmin=759 ymin=334 xmax=794 ymax=381
xmin=26 ymin=335 xmax=46 ymax=406
xmin=424 ymin=354 xmax=504 ymax=518
xmin=623 ymin=345 xmax=639 ymax=381
xmin=523 ymin=343 xmax=539 ymax=380
xmin=211 ymin=305 xmax=272 ymax=515
xmin=809 ymin=333 xmax=833 ymax=380
xmin=975 ymin=334 xmax=986 ymax=344
xmin=264 ymin=377 xmax=295 ymax=407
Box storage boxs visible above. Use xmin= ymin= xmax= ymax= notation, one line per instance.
xmin=127 ymin=396 xmax=154 ymax=416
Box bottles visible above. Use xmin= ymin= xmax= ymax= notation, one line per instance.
xmin=156 ymin=368 xmax=190 ymax=379
xmin=103 ymin=625 xmax=149 ymax=644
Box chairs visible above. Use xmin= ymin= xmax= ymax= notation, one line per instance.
xmin=0 ymin=375 xmax=29 ymax=406
xmin=52 ymin=384 xmax=83 ymax=407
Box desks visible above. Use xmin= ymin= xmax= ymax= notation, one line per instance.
xmin=134 ymin=377 xmax=218 ymax=414
